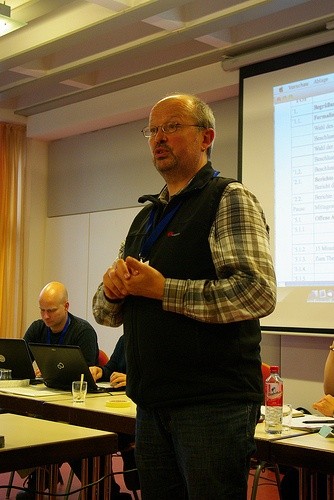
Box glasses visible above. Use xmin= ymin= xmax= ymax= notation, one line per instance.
xmin=141 ymin=122 xmax=207 ymax=137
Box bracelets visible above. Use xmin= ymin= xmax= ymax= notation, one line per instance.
xmin=329 ymin=346 xmax=334 ymax=351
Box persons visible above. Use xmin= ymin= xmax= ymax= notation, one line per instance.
xmin=88 ymin=334 xmax=127 ymax=390
xmin=92 ymin=94 xmax=276 ymax=500
xmin=15 ymin=282 xmax=120 ymax=500
xmin=312 ymin=339 xmax=334 ymax=417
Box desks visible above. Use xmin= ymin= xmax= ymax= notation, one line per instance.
xmin=1 ymin=381 xmax=334 ymax=500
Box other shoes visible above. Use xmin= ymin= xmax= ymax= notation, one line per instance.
xmin=16 ymin=469 xmax=64 ymax=500
xmin=78 ymin=483 xmax=120 ymax=500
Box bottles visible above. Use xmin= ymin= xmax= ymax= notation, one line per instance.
xmin=264 ymin=366 xmax=283 ymax=434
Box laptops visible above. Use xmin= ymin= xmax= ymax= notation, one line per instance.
xmin=27 ymin=342 xmax=126 ymax=393
xmin=0 ymin=338 xmax=41 ymax=380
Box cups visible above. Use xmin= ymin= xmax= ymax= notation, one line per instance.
xmin=72 ymin=381 xmax=87 ymax=402
xmin=0 ymin=369 xmax=12 ymax=380
